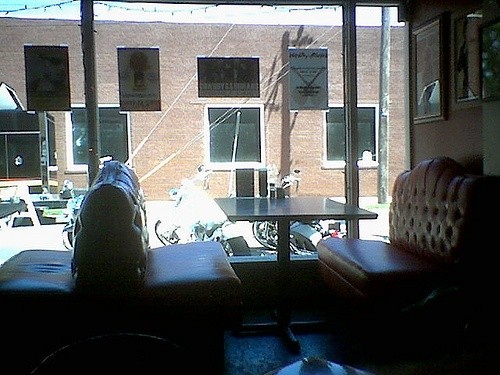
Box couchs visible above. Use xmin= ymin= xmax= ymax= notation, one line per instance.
xmin=0 ymin=161 xmax=113 ymax=353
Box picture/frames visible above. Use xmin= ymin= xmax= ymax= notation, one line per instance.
xmin=478 ymin=16 xmax=500 ymax=102
xmin=454 ymin=5 xmax=483 ymax=103
xmin=410 ymin=13 xmax=448 ymax=125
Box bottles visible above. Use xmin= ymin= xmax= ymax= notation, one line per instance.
xmin=270 ymin=183 xmax=275 ymax=198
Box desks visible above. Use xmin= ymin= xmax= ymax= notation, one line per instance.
xmin=218 ymin=198 xmax=378 ymax=354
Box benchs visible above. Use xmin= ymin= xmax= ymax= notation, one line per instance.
xmin=109 ymin=160 xmax=241 ymax=353
xmin=317 ymin=157 xmax=500 ymax=334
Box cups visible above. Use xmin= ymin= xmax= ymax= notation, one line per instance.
xmin=277 ymin=188 xmax=284 ymax=198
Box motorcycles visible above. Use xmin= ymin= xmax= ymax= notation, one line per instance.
xmin=251 ymin=168 xmax=348 ymax=255
xmin=60 ymin=180 xmax=88 ymax=250
xmin=152 ymin=164 xmax=252 ymax=257
